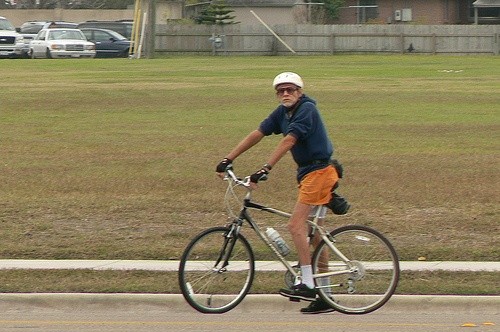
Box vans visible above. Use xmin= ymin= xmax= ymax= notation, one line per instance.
xmin=17 ymin=20 xmax=47 ymax=59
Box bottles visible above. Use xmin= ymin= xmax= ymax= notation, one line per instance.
xmin=266 ymin=227 xmax=292 ymax=256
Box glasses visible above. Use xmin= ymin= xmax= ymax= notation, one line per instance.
xmin=277 ymin=87 xmax=298 ymax=95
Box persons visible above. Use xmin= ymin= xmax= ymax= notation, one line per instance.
xmin=216 ymin=71 xmax=339 ymax=315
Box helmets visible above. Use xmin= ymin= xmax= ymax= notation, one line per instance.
xmin=273 ymin=72 xmax=303 ymax=90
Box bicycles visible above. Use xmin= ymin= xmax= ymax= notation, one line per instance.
xmin=175 ymin=158 xmax=401 ymax=316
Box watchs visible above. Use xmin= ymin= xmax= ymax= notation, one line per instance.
xmin=264 ymin=163 xmax=272 ymax=170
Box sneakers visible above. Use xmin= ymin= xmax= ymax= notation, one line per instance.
xmin=279 ymin=283 xmax=316 ymax=301
xmin=300 ymin=296 xmax=335 ymax=313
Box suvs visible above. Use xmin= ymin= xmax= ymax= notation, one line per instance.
xmin=0 ymin=16 xmax=25 ymax=59
xmin=74 ymin=18 xmax=134 ymax=41
xmin=41 ymin=19 xmax=80 ymax=30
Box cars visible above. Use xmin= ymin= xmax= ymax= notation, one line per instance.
xmin=27 ymin=27 xmax=97 ymax=59
xmin=79 ymin=28 xmax=135 ymax=59
